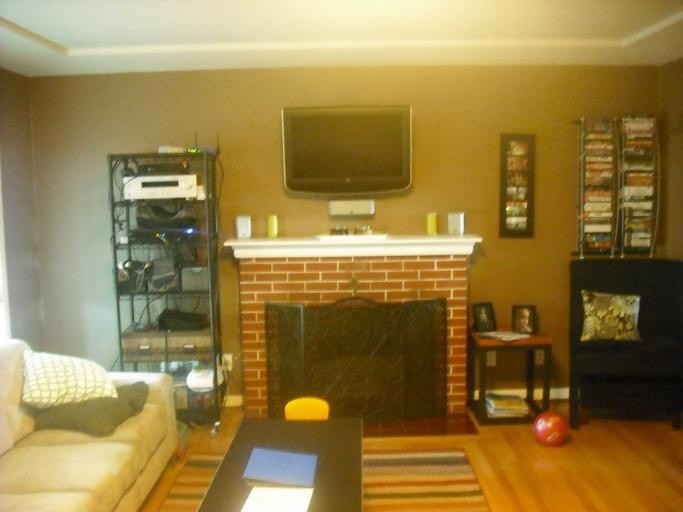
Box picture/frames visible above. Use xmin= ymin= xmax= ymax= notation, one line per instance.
xmin=512 ymin=304 xmax=536 ymax=334
xmin=471 ymin=302 xmax=496 ymax=332
xmin=499 ymin=134 xmax=536 ymax=238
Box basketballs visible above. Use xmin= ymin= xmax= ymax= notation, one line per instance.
xmin=533 ymin=411 xmax=569 ymax=447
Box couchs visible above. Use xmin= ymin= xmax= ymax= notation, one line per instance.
xmin=568 ymin=258 xmax=683 ymax=430
xmin=0 ymin=339 xmax=178 ymax=512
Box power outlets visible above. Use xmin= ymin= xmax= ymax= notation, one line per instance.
xmin=222 ymin=353 xmax=233 ymax=372
xmin=486 ymin=350 xmax=496 ymax=367
xmin=534 ymin=349 xmax=544 ymax=368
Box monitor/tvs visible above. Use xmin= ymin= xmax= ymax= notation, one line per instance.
xmin=281 ymin=104 xmax=413 ymax=199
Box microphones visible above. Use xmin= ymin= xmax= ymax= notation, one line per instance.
xmin=158 ymin=145 xmax=201 ymax=154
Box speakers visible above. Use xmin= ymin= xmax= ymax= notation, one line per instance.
xmin=235 ymin=215 xmax=252 ymax=240
xmin=447 ymin=211 xmax=464 ymax=236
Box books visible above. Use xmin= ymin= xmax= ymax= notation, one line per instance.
xmin=487 ymin=393 xmax=529 ymax=419
xmin=579 ymin=116 xmax=616 ymax=250
xmin=480 ymin=330 xmax=531 ymax=342
xmin=620 ymin=113 xmax=657 ymax=249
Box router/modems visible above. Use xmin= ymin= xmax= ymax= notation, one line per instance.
xmin=193 ymin=130 xmax=219 ymax=155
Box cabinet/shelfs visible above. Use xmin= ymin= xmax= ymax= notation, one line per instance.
xmin=570 ymin=110 xmax=619 ymax=261
xmin=621 ymin=115 xmax=661 ymax=259
xmin=107 ymin=151 xmax=228 ymax=433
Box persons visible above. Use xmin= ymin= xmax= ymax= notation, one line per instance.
xmin=514 ymin=306 xmax=531 ymax=332
xmin=479 ymin=307 xmax=488 ymax=329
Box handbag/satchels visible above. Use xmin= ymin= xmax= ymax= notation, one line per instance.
xmin=122 ymin=257 xmax=153 ymax=294
xmin=147 ymin=272 xmax=180 ymax=293
xmin=180 ymin=266 xmax=210 ymax=293
xmin=155 ymin=308 xmax=207 ymax=331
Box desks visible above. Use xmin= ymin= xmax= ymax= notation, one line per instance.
xmin=466 ymin=331 xmax=553 ymax=425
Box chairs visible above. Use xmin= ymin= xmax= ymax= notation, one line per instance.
xmin=285 ymin=397 xmax=330 ymax=421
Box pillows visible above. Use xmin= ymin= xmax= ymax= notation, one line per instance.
xmin=34 ymin=381 xmax=149 ymax=438
xmin=580 ymin=288 xmax=643 ymax=343
xmin=21 ymin=350 xmax=118 ymax=409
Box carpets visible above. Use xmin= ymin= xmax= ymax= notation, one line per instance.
xmin=363 ymin=413 xmax=480 ymax=438
xmin=157 ymin=446 xmax=489 ymax=512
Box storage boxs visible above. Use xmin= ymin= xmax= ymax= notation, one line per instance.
xmin=167 ymin=326 xmax=218 ymax=360
xmin=121 ymin=321 xmax=167 ymax=362
xmin=181 ymin=262 xmax=218 ymax=292
